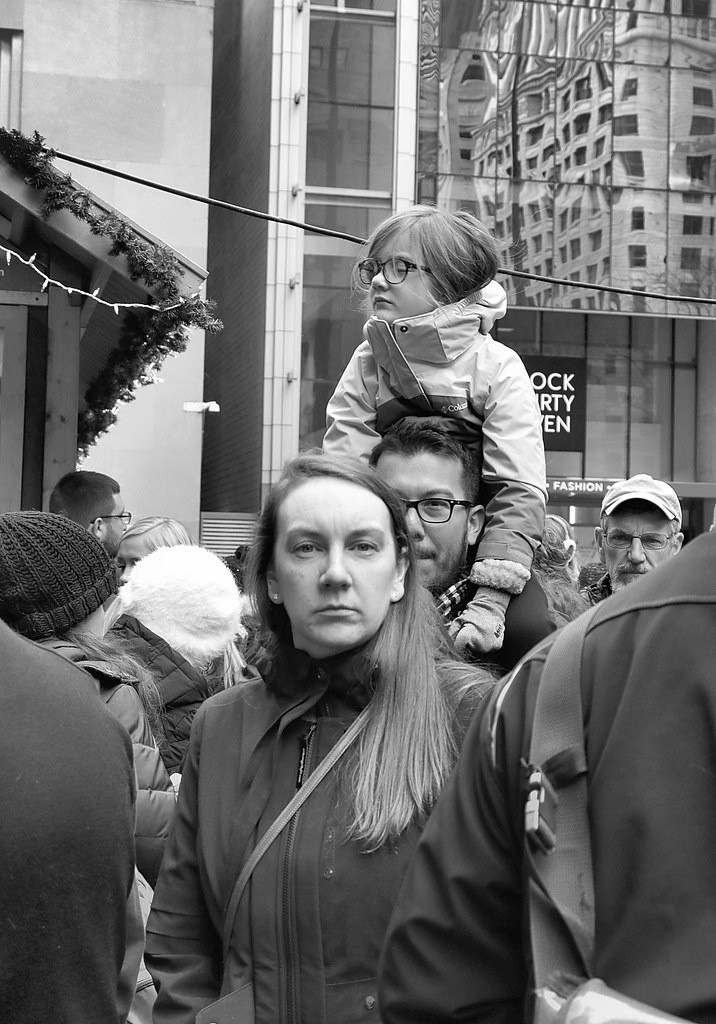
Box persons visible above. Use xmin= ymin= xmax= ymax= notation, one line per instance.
xmin=4 ymin=205 xmax=716 ymax=1021
